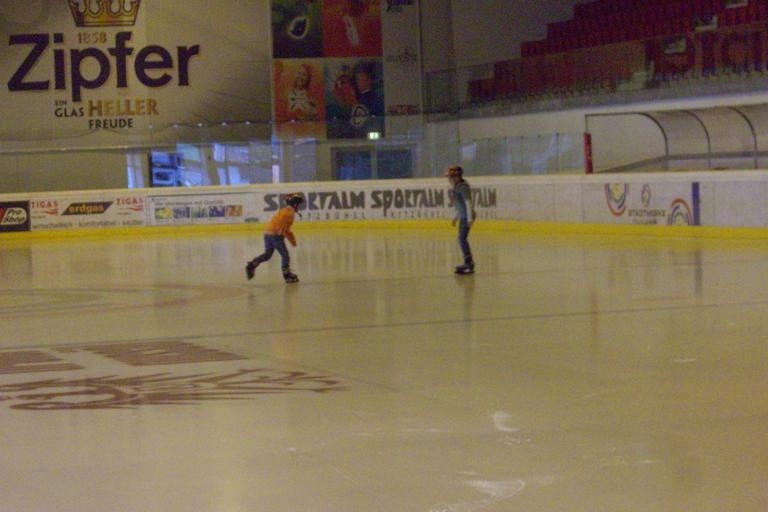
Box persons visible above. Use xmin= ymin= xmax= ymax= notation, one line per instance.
xmin=274 ymin=60 xmax=316 ymax=121
xmin=333 ymin=59 xmax=384 ymax=180
xmin=444 ymin=166 xmax=476 ymax=275
xmin=244 ymin=194 xmax=304 ymax=283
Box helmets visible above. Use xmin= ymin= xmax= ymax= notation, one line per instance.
xmin=286 ymin=194 xmax=303 ymax=205
xmin=445 ymin=166 xmax=464 ymax=176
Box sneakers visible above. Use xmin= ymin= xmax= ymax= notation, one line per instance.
xmin=284 ymin=271 xmax=297 ymax=280
xmin=247 ymin=261 xmax=255 ymax=278
xmin=457 ymin=260 xmax=473 ymax=270
